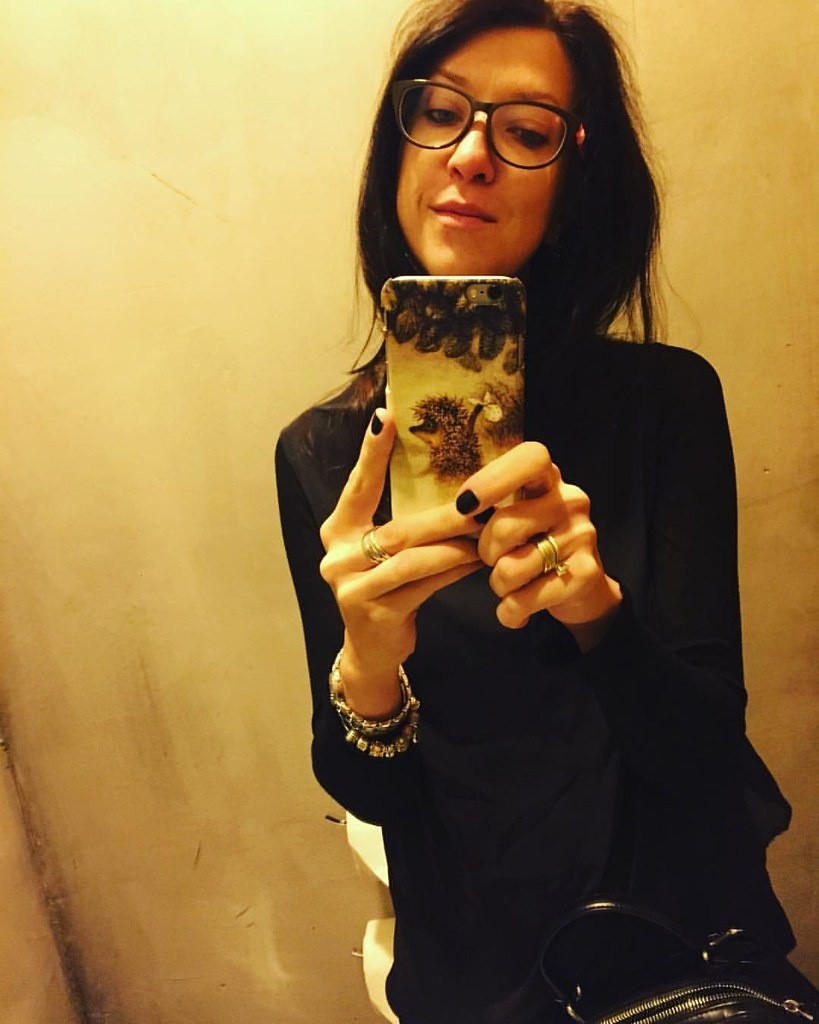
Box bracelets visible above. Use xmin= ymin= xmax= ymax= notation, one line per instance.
xmin=337 ymin=697 xmax=420 ymax=756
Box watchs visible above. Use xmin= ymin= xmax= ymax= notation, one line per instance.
xmin=326 ymin=645 xmax=412 ymax=732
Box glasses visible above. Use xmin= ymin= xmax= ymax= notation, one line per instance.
xmin=390 ymin=79 xmax=585 ymax=169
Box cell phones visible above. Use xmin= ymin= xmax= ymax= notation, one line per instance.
xmin=379 ymin=274 xmax=528 ymax=541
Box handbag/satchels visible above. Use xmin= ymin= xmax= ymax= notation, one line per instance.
xmin=537 ymin=894 xmax=819 ymax=1024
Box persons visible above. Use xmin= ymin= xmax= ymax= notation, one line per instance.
xmin=274 ymin=0 xmax=819 ymax=1024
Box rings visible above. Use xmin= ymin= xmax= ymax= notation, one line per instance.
xmin=529 ymin=539 xmax=556 ymax=573
xmin=548 ymin=536 xmax=569 ymax=576
xmin=361 ymin=526 xmax=390 ymax=566
